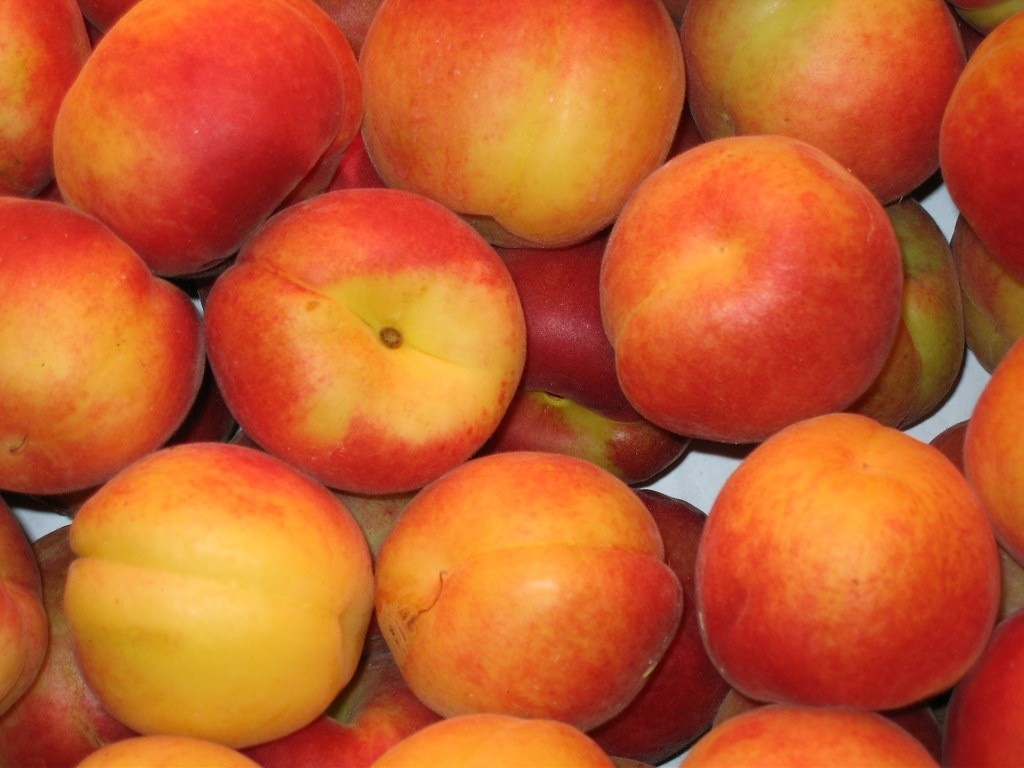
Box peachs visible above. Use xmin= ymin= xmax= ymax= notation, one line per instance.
xmin=1 ymin=0 xmax=1023 ymax=768
